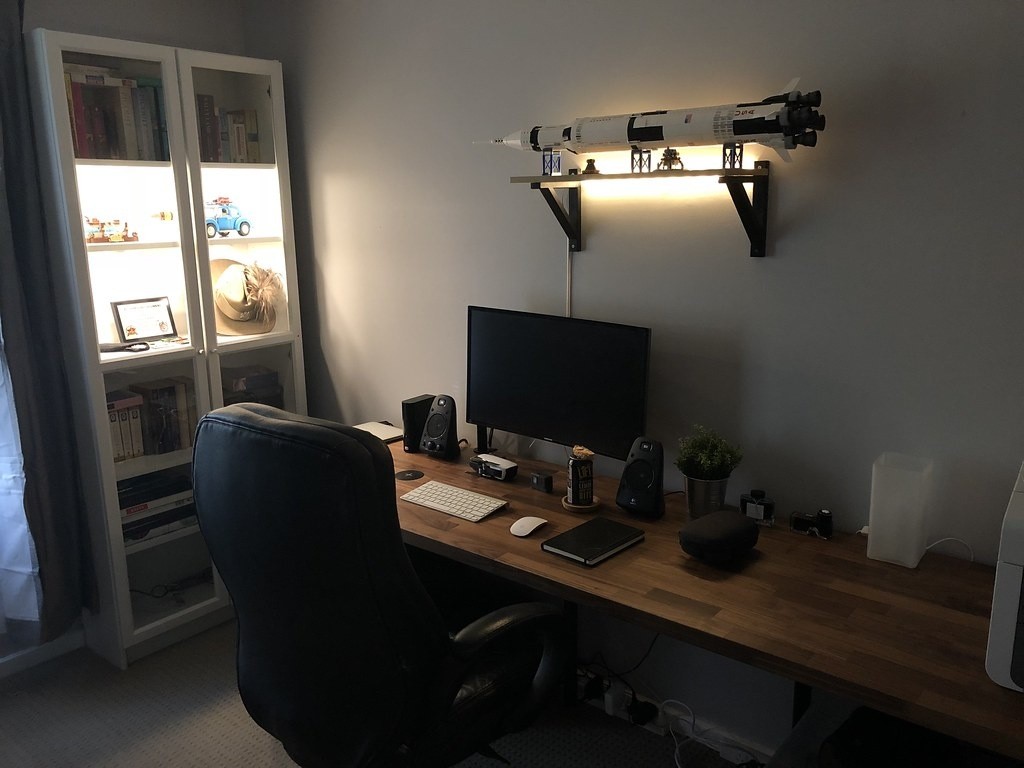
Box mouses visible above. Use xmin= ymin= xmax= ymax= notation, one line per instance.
xmin=510 ymin=516 xmax=549 ymax=539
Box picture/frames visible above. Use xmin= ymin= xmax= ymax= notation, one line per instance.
xmin=109 ymin=296 xmax=178 ymax=344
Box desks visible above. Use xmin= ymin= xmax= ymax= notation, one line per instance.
xmin=387 ymin=426 xmax=1024 ymax=762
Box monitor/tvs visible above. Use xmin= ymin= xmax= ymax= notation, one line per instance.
xmin=462 ymin=305 xmax=652 ymax=467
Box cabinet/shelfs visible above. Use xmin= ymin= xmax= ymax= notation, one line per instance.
xmin=25 ymin=26 xmax=310 ymax=671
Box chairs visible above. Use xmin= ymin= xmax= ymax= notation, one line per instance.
xmin=190 ymin=400 xmax=578 ymax=768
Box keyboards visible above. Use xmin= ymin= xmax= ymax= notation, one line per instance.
xmin=401 ymin=480 xmax=509 ymax=523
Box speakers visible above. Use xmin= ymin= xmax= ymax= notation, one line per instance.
xmin=420 ymin=394 xmax=462 ymax=462
xmin=613 ymin=436 xmax=667 ymax=525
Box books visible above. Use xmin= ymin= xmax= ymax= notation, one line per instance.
xmin=541 ymin=516 xmax=645 ymax=567
xmin=117 ymin=464 xmax=199 ymax=548
xmin=220 ymin=364 xmax=286 ymax=410
xmin=62 ymin=61 xmax=169 ymax=161
xmin=105 ymin=375 xmax=197 ymax=462
xmin=194 ymin=93 xmax=261 ymax=163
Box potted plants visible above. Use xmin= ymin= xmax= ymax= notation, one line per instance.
xmin=672 ymin=424 xmax=745 ymax=519
